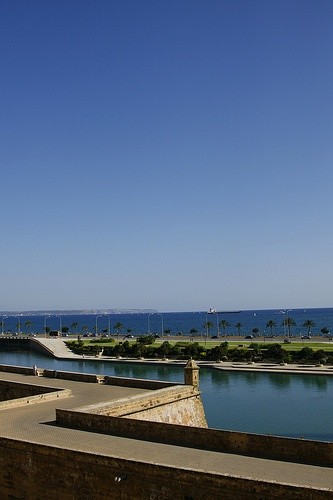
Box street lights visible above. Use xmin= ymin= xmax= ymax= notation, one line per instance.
xmin=105 ymin=315 xmax=110 ymax=336
xmin=205 ymin=311 xmax=211 ymax=337
xmin=147 ymin=313 xmax=154 ymax=333
xmin=158 ymin=314 xmax=164 ymax=336
xmin=213 ymin=312 xmax=219 ymax=337
xmin=287 ymin=309 xmax=292 ymax=337
xmin=43 ymin=316 xmax=51 ymax=338
xmin=96 ymin=315 xmax=102 ymax=334
xmin=2 ymin=316 xmax=8 ymax=333
xmin=55 ymin=315 xmax=62 ymax=332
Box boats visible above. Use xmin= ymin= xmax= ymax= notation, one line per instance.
xmin=208 ymin=307 xmax=217 ymax=314
